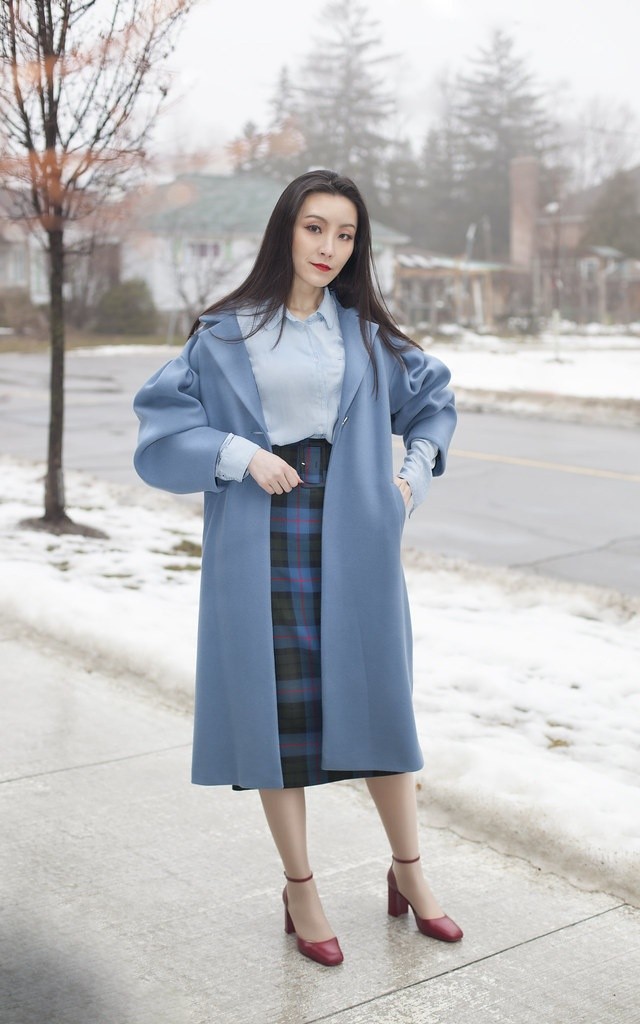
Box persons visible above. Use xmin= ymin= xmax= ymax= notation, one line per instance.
xmin=134 ymin=169 xmax=463 ymax=967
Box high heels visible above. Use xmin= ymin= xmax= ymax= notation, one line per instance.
xmin=387 ymin=854 xmax=463 ymax=942
xmin=283 ymin=871 xmax=344 ymax=966
